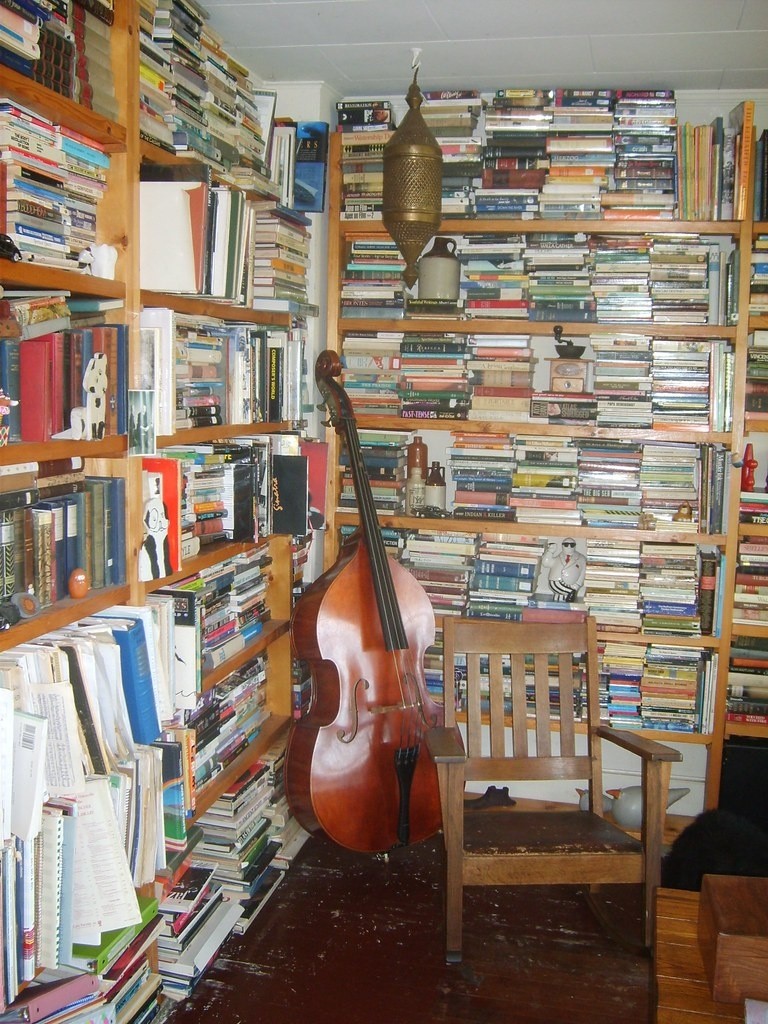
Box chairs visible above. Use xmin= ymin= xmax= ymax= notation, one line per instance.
xmin=424 ymin=616 xmax=683 ymax=963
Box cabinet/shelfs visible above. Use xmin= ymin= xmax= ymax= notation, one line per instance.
xmin=0 ymin=0 xmax=768 ymax=1024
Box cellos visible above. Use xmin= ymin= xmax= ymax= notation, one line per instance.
xmin=285 ymin=349 xmax=450 ymax=854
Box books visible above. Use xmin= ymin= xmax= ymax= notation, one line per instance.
xmin=0 ymin=0 xmax=768 ymax=1024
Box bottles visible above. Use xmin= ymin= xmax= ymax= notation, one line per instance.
xmin=404 ymin=436 xmax=446 ymax=516
xmin=418 ymin=237 xmax=461 ymax=308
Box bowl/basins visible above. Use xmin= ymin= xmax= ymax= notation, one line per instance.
xmin=554 ymin=344 xmax=586 ymax=359
